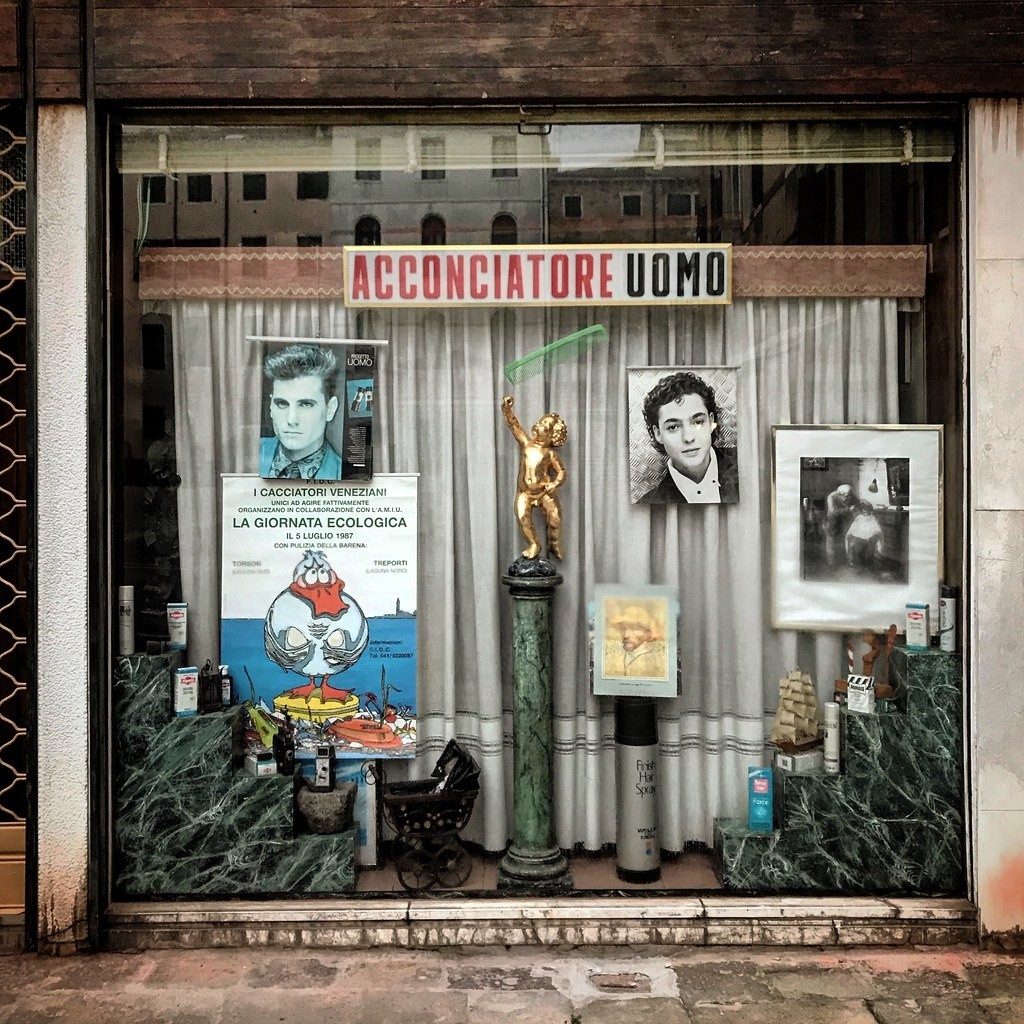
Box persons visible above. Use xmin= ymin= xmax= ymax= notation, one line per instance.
xmin=826 ymin=483 xmax=886 ymax=573
xmin=608 ymin=605 xmax=664 ymax=678
xmin=635 ymin=372 xmax=738 ymax=505
xmin=501 ymin=396 xmax=567 ymax=560
xmin=259 ymin=344 xmax=341 ymax=481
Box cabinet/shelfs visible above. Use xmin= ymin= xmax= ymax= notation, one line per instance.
xmin=114 ymin=646 xmax=359 ymax=894
xmin=713 ymin=643 xmax=968 ymax=898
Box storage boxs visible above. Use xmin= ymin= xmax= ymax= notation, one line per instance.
xmin=245 ymin=755 xmax=277 ymax=776
xmin=174 ymin=667 xmax=199 ymax=716
xmin=777 ymin=751 xmax=823 ymax=772
xmin=167 ymin=603 xmax=188 ymax=649
xmin=748 ymin=766 xmax=773 ymax=831
xmin=848 ymin=674 xmax=875 ymax=714
xmin=906 ymin=603 xmax=930 ymax=651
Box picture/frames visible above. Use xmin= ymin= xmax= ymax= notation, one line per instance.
xmin=771 ymin=425 xmax=943 ymax=636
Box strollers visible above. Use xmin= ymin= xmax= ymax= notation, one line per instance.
xmin=384 ymin=739 xmax=480 ymax=888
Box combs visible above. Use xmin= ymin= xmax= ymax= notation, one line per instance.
xmin=504 ymin=323 xmax=609 ymax=387
